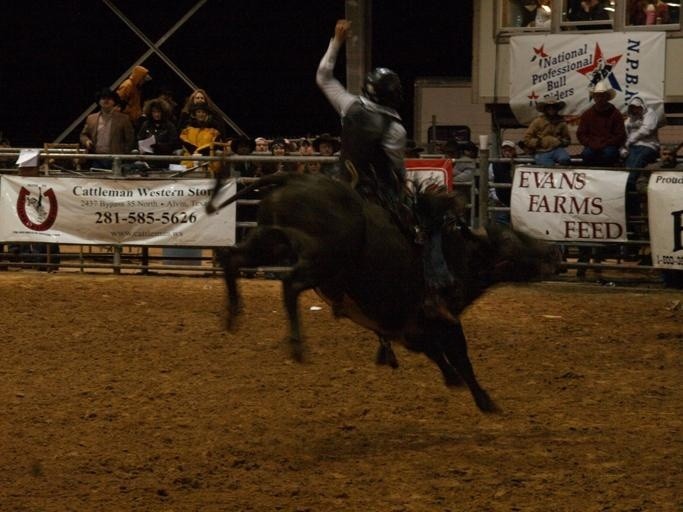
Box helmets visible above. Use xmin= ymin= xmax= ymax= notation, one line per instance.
xmin=361 ymin=65 xmax=404 ymax=109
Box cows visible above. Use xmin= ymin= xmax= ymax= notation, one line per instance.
xmin=203 ymin=157 xmax=563 ymax=414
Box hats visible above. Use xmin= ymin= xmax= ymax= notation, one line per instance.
xmin=298 ymin=131 xmax=342 ymax=153
xmin=623 ymin=96 xmax=647 ymax=110
xmin=93 ymin=87 xmax=121 ymax=105
xmin=500 ymin=140 xmax=517 ymax=148
xmin=588 ymin=80 xmax=617 ymax=100
xmin=270 ymin=138 xmax=287 ymax=149
xmin=534 ymin=94 xmax=567 ymax=113
xmin=231 ymin=136 xmax=256 ymax=153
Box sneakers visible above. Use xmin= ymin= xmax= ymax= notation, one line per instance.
xmin=426 ymin=301 xmax=460 ymax=329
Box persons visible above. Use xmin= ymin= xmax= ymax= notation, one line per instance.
xmin=77 ymin=65 xmax=223 ymax=175
xmin=314 ymin=18 xmax=458 ymax=324
xmin=520 ymin=96 xmax=571 ymax=167
xmin=513 ymin=0 xmax=667 ymax=29
xmin=576 ymin=82 xmax=625 ymax=168
xmin=633 ymin=144 xmax=683 ymax=232
xmin=615 ymin=92 xmax=659 ymax=189
